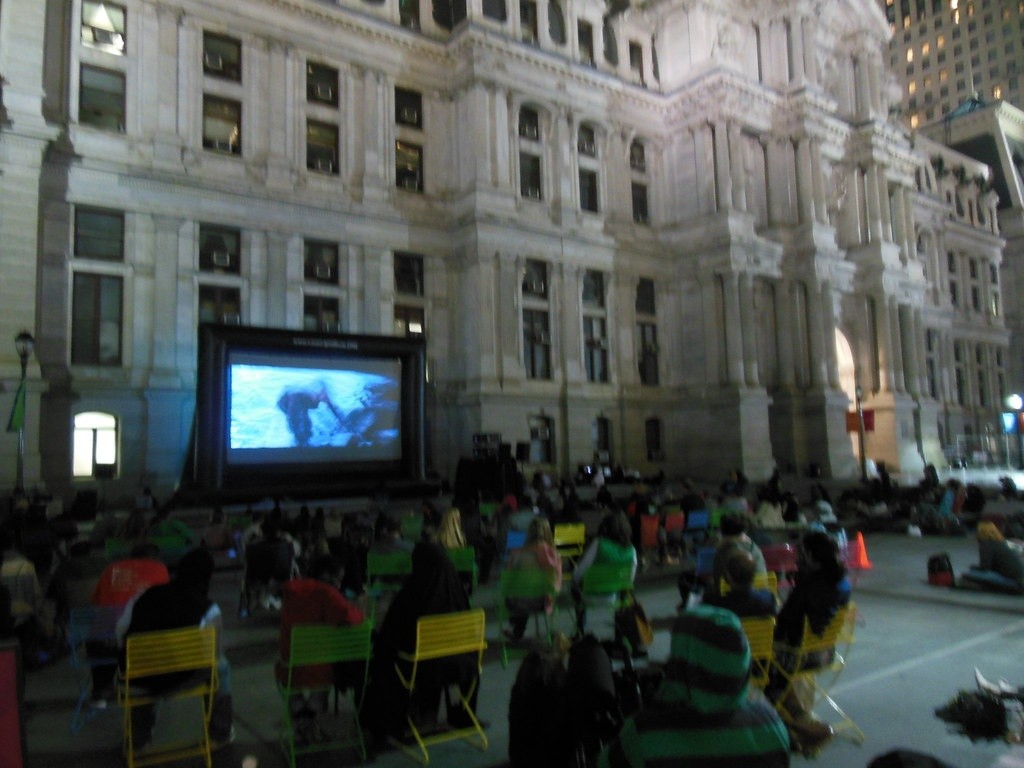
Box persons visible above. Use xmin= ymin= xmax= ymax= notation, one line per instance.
xmin=0 ymin=435 xmax=1024 ymax=768
xmin=278 ymin=377 xmax=400 ymax=448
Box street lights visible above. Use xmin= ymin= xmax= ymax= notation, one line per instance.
xmin=1008 ymin=392 xmax=1023 ymax=469
xmin=12 ymin=330 xmax=37 ymax=506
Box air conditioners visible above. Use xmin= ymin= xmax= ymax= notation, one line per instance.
xmin=594 ymin=339 xmax=609 ymax=349
xmin=315 ymin=158 xmax=332 ymax=172
xmin=322 ymin=322 xmax=340 ymax=333
xmin=534 ymin=333 xmax=551 ymax=344
xmin=522 ymin=124 xmax=537 ymax=137
xmin=311 ymin=264 xmax=330 ymax=278
xmin=313 ymin=84 xmax=334 ymax=103
xmin=222 ymin=312 xmax=239 ymax=324
xmin=528 ymin=281 xmax=545 ymax=294
xmin=213 ymin=138 xmax=231 ymax=153
xmin=531 ymin=427 xmax=549 ymax=440
xmin=523 ymin=188 xmax=540 ymax=198
xmin=578 ymin=143 xmax=595 ymax=154
xmin=401 ymin=178 xmax=417 ymax=191
xmin=594 ymin=450 xmax=609 ymax=462
xmin=211 ymin=252 xmax=229 ymax=267
xmin=91 ymin=27 xmax=111 ymax=44
xmin=400 ymin=107 xmax=417 ymax=124
xmin=203 ymin=50 xmax=223 ymax=73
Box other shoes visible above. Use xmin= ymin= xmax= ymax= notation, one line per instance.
xmin=92 ymin=699 xmax=106 ymax=713
xmin=209 ymin=724 xmax=235 ymax=750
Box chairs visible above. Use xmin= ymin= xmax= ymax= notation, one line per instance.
xmin=69 ymin=607 xmax=132 ymax=734
xmin=274 ymin=615 xmax=376 ymax=768
xmin=117 ymin=626 xmax=220 ymax=768
xmin=227 ymin=516 xmax=252 ymax=543
xmin=103 ymin=521 xmax=183 ymax=562
xmin=644 ymin=507 xmax=799 ymax=599
xmin=0 ymin=574 xmax=36 ymax=615
xmin=844 ymin=532 xmax=871 ymax=590
xmin=365 ymin=496 xmax=635 ymax=669
xmin=742 ymin=605 xmax=865 ymax=760
xmin=389 ymin=608 xmax=489 ymax=764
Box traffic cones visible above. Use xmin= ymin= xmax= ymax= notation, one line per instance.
xmin=849 ymin=531 xmax=874 ymax=572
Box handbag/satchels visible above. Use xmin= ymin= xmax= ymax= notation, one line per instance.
xmin=928 ymin=552 xmax=956 ymax=589
xmin=614 ymin=587 xmax=653 ymax=648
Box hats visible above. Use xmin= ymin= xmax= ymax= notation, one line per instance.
xmin=814 ymin=499 xmax=838 ymax=524
